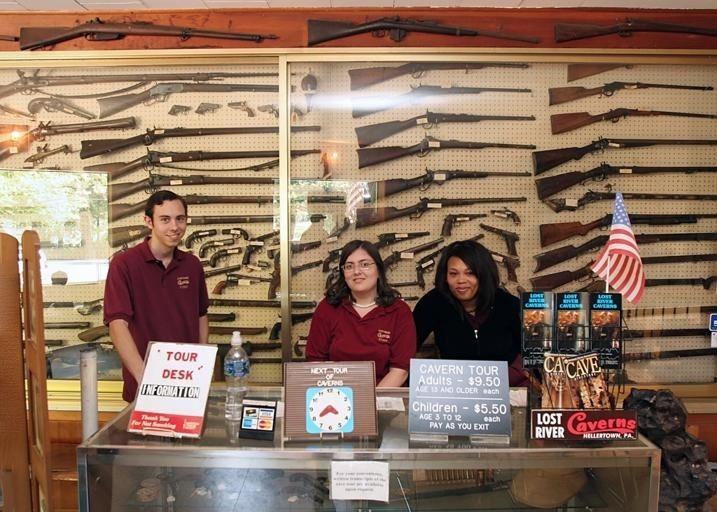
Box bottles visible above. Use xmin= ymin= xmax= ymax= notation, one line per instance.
xmin=223 ymin=337 xmax=250 ymax=420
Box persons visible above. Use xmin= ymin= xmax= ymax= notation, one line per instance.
xmin=300 ymin=213 xmax=331 ymax=243
xmin=103 ymin=190 xmax=212 ymax=403
xmin=413 ymin=239 xmax=531 ymax=388
xmin=304 ymin=239 xmax=417 ymax=387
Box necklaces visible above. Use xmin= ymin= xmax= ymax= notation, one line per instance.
xmin=352 ymin=301 xmax=377 ymax=307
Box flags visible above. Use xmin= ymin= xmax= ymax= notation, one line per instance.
xmin=589 ymin=190 xmax=646 ymax=305
xmin=344 ymin=182 xmax=370 ymax=221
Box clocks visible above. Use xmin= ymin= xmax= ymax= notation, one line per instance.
xmin=282 ymin=360 xmax=379 ymax=444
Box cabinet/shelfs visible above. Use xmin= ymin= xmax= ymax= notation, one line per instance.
xmin=73 ymin=388 xmax=661 ymax=512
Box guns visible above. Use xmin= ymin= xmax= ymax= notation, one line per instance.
xmin=0 ymin=15 xmax=717 ymax=381
xmin=206 ymin=468 xmax=251 ymax=494
xmin=282 ymin=485 xmax=324 ymax=505
xmin=236 ymin=468 xmax=281 ymax=496
xmin=369 ymin=477 xmax=511 ymax=506
xmin=289 ymin=471 xmax=329 ymax=495
xmin=177 ymin=471 xmax=227 ymax=499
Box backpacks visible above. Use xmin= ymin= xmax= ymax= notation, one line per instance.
xmin=622 ymin=387 xmax=715 ymax=512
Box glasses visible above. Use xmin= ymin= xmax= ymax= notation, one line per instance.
xmin=340 ymin=262 xmax=377 ymax=271
